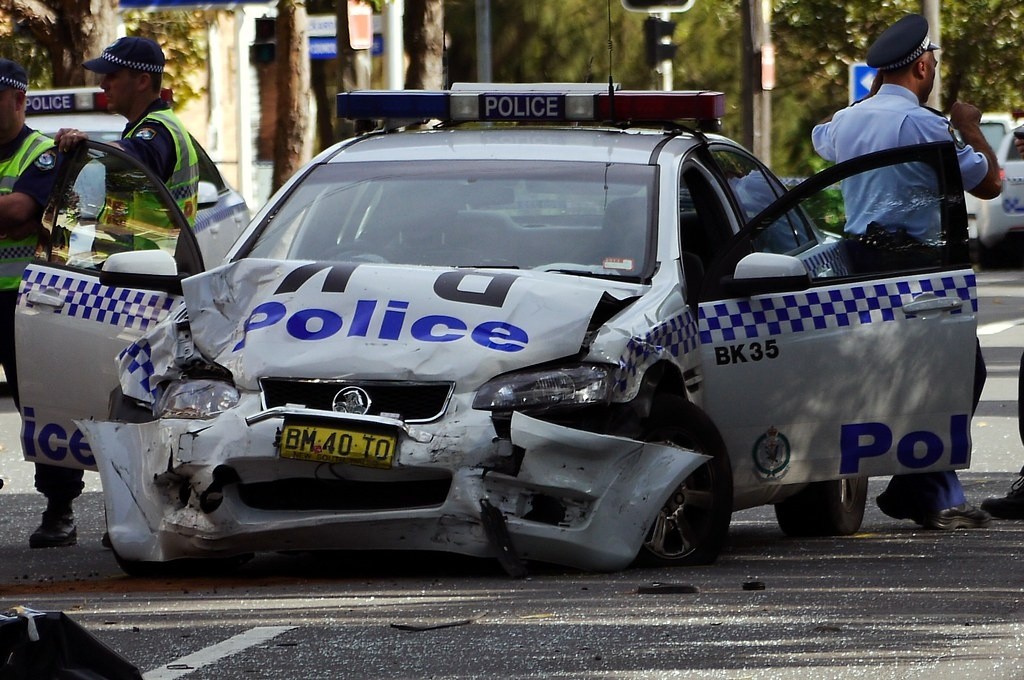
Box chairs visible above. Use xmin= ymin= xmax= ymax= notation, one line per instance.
xmin=343 ymin=184 xmax=704 ymax=290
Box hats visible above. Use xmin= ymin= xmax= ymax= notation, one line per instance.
xmin=865 ymin=14 xmax=941 ymax=71
xmin=81 ymin=36 xmax=165 ymax=76
xmin=0 ymin=57 xmax=28 ymax=92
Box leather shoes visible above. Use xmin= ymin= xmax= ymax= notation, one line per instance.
xmin=29 ymin=501 xmax=112 ymax=548
xmin=877 ymin=482 xmax=1024 ymax=529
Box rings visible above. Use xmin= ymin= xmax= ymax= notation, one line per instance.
xmin=71 ymin=132 xmax=78 ymax=136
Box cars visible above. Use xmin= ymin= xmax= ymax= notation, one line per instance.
xmin=13 ymin=82 xmax=981 ymax=577
xmin=943 ymin=112 xmax=1023 ymax=270
xmin=25 ymin=86 xmax=251 ymax=274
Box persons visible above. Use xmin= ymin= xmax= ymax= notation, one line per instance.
xmin=981 ymin=120 xmax=1023 ymax=520
xmin=53 ymin=37 xmax=200 ymax=545
xmin=809 ymin=15 xmax=1003 ymax=532
xmin=0 ymin=58 xmax=85 ymax=548
